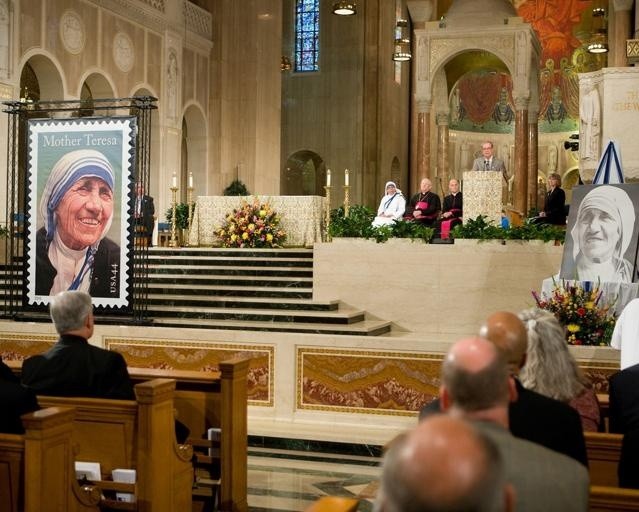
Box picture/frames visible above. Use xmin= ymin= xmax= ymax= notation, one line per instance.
xmin=22 ymin=115 xmax=137 ymax=315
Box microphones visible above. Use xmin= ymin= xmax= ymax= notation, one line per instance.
xmin=484 ymin=160 xmax=487 ymax=171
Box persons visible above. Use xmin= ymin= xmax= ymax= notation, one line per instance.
xmin=405 ymin=178 xmax=442 ymax=227
xmin=436 ymin=179 xmax=463 ymax=228
xmin=514 ymin=308 xmax=606 ymax=433
xmin=470 ymin=142 xmax=515 ymax=186
xmin=570 ymin=185 xmax=639 ymax=284
xmin=530 ymin=174 xmax=566 ymax=225
xmin=607 ymin=364 xmax=638 ymax=490
xmin=35 ymin=149 xmax=120 ymax=295
xmin=23 ymin=290 xmax=192 ymax=445
xmin=374 ymin=413 xmax=517 ymax=511
xmin=438 ymin=336 xmax=591 ymax=512
xmin=133 ymin=181 xmax=155 ymax=247
xmin=610 ymin=298 xmax=639 ymax=371
xmin=371 ymin=181 xmax=407 ymax=226
xmin=419 ymin=310 xmax=589 ymax=469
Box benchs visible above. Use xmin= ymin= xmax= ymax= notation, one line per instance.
xmin=581 ymin=393 xmax=639 ymax=511
xmin=0 ymin=359 xmax=249 ymax=512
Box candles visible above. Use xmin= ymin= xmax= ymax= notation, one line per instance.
xmin=345 ymin=168 xmax=349 ymax=185
xmin=172 ymin=171 xmax=176 ymax=186
xmin=189 ymin=172 xmax=193 ymax=187
xmin=326 ymin=169 xmax=330 ymax=187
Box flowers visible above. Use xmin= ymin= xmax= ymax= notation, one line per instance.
xmin=211 ymin=192 xmax=287 ymax=249
xmin=532 ymin=275 xmax=619 ymax=345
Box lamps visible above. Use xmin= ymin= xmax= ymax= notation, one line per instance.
xmin=392 ymin=19 xmax=411 ymax=62
xmin=333 ymin=0 xmax=357 ymax=16
xmin=588 ymin=8 xmax=608 ymax=54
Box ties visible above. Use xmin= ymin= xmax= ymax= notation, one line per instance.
xmin=486 ymin=161 xmax=490 ymax=171
